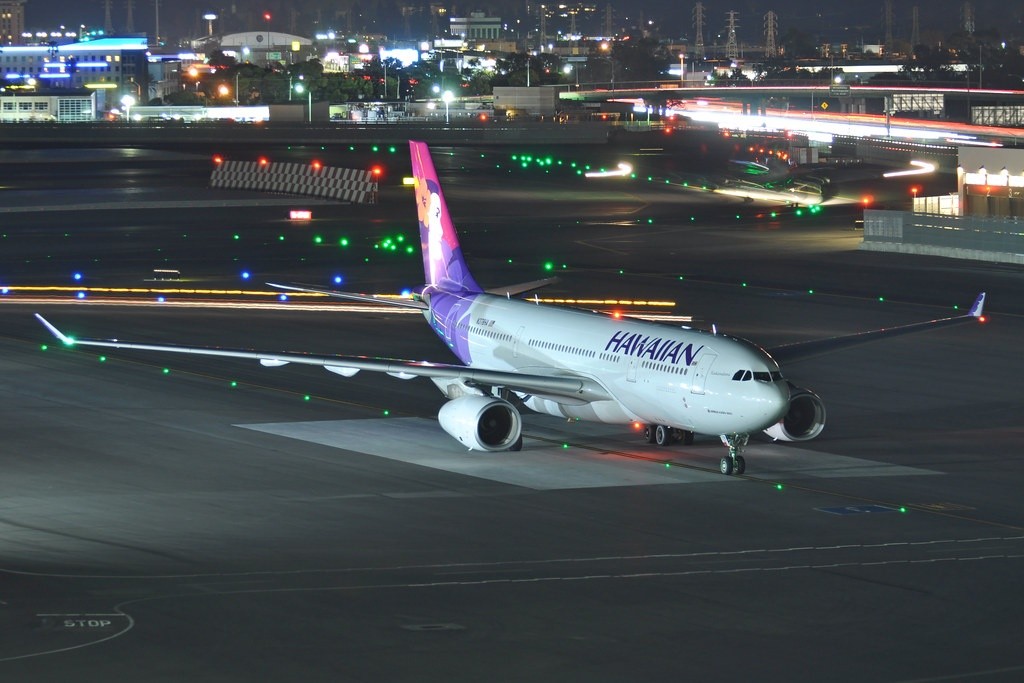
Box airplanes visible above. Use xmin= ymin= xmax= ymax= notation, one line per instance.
xmin=33 ymin=138 xmax=988 ymax=477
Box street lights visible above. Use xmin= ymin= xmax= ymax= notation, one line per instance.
xmin=239 ymin=43 xmax=250 ymax=62
xmin=264 ymin=14 xmax=272 ymax=66
xmin=381 ymin=63 xmax=387 ymax=99
xmin=679 ymin=53 xmax=684 ymax=89
xmin=862 ymin=196 xmax=870 ymax=210
xmin=564 ymin=61 xmax=580 ymax=91
xmin=443 ymin=90 xmax=454 ymax=123
xmin=235 ymin=71 xmax=242 ymax=106
xmin=189 ymin=68 xmax=199 ymax=97
xmin=295 ymin=86 xmax=313 ymax=122
xmin=911 ymin=189 xmax=918 ymax=199
xmin=122 ymin=96 xmax=134 ymax=122
